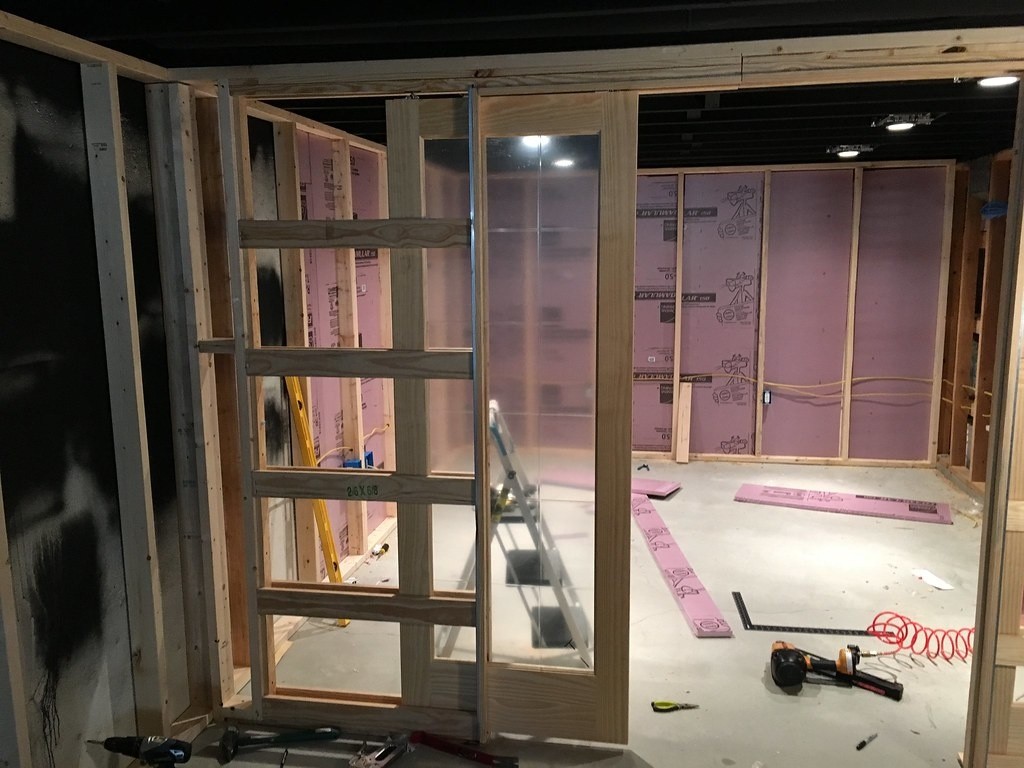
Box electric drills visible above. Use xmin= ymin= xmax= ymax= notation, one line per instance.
xmin=771 ymin=640 xmax=859 ymax=686
xmin=86 ymin=736 xmax=192 ymax=768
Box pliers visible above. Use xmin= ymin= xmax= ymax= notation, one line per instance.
xmin=651 ymin=701 xmax=699 ymax=712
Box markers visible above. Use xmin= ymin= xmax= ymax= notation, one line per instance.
xmin=857 ymin=734 xmax=877 ymax=749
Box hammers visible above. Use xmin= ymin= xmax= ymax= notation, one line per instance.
xmin=220 ymin=724 xmax=339 ymax=761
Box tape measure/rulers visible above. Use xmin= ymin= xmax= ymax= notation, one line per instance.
xmin=733 ymin=592 xmax=896 ymax=639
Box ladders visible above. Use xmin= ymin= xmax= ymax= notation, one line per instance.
xmin=434 ymin=400 xmax=593 ymax=666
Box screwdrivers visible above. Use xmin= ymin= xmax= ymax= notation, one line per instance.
xmin=377 ymin=544 xmax=389 ymax=560
